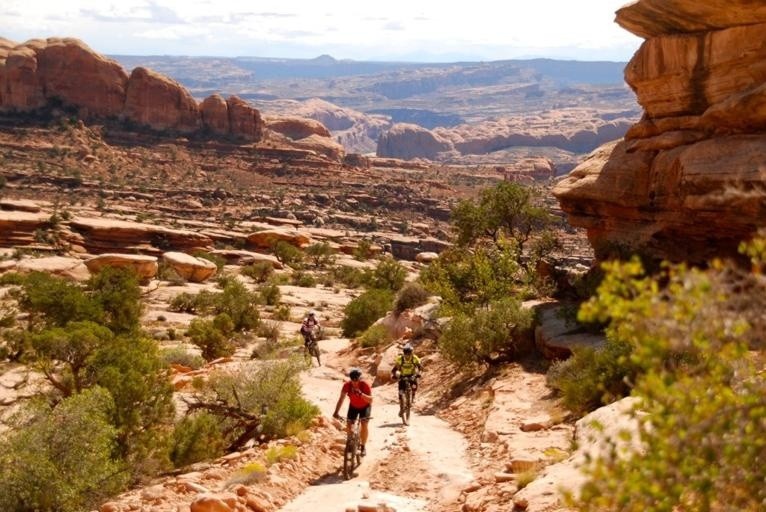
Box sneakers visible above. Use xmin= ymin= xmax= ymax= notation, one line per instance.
xmin=359 ymin=444 xmax=366 ymax=457
xmin=411 ymin=397 xmax=416 ymax=403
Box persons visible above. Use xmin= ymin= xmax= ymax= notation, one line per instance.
xmin=332 ymin=369 xmax=373 ymax=458
xmin=391 ymin=346 xmax=425 ymax=416
xmin=300 ymin=313 xmax=322 ymax=354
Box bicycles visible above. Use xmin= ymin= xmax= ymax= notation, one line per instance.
xmin=335 ymin=417 xmax=372 ymax=479
xmin=304 ymin=335 xmax=321 ymax=366
xmin=392 ymin=377 xmax=418 ymax=426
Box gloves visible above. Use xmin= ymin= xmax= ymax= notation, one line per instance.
xmin=353 ymin=389 xmax=363 ymax=399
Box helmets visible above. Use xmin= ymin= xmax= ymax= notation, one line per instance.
xmin=349 ymin=369 xmax=362 ymax=380
xmin=308 ymin=311 xmax=315 ymax=317
xmin=403 ymin=347 xmax=413 ymax=354
